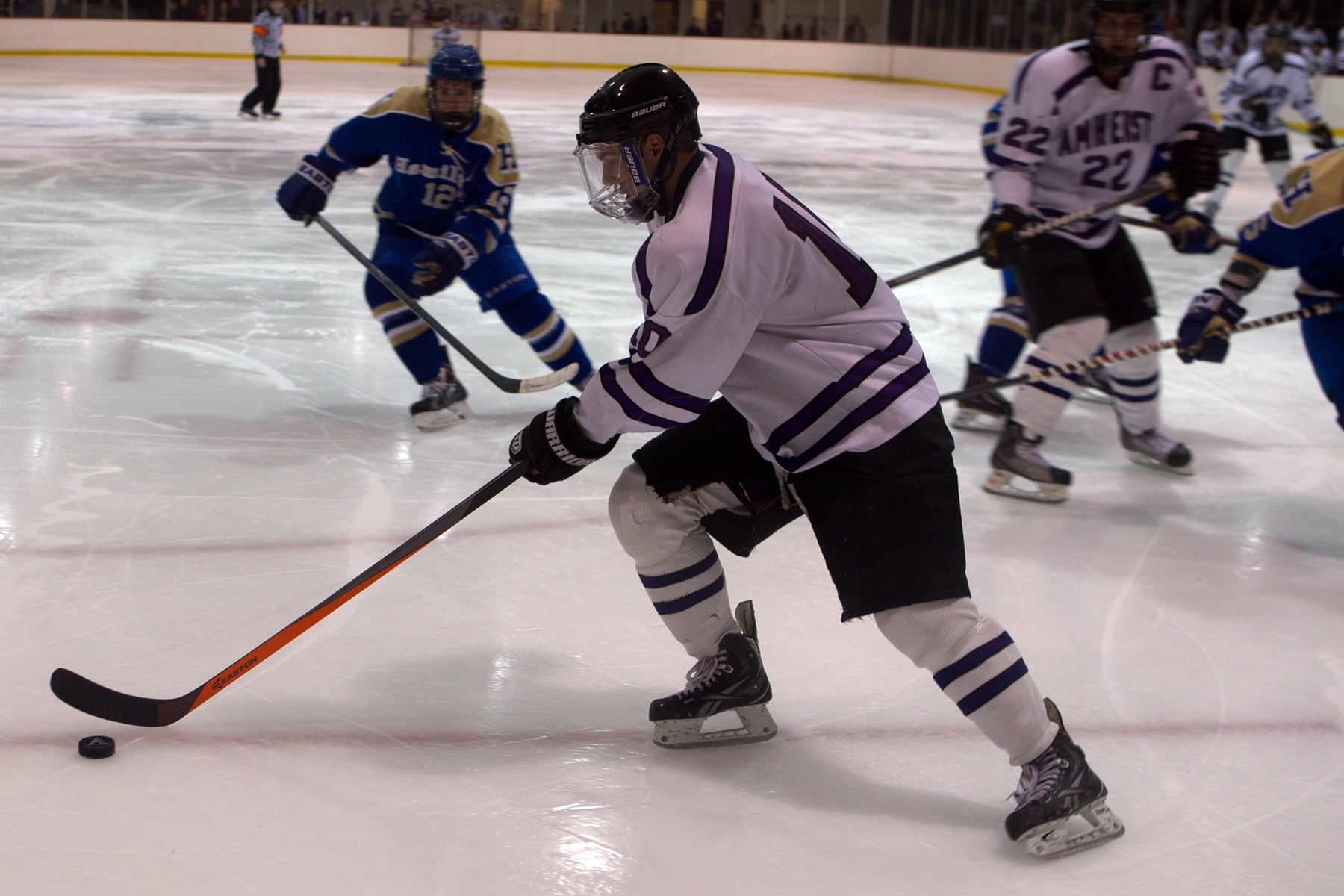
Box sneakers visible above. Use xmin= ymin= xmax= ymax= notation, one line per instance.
xmin=1080 ymin=358 xmax=1115 ymax=401
xmin=1106 ymin=400 xmax=1196 ymax=475
xmin=240 ymin=106 xmax=258 ymax=120
xmin=261 ymin=109 xmax=280 ymax=120
xmin=983 ymin=402 xmax=1072 ymax=503
xmin=648 ymin=599 xmax=777 ymax=749
xmin=409 ymin=345 xmax=475 ymax=428
xmin=953 ymin=354 xmax=1020 ymax=435
xmin=1004 ymin=697 xmax=1124 ymax=859
xmin=576 ymin=369 xmax=595 ymax=392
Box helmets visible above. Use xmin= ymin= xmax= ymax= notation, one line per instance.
xmin=573 ymin=63 xmax=698 ymax=226
xmin=1260 ymin=24 xmax=1292 ymax=62
xmin=1081 ymin=0 xmax=1151 ymax=70
xmin=425 ymin=45 xmax=487 ymax=131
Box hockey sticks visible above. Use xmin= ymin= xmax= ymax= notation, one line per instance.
xmin=939 ymin=302 xmax=1343 ymax=403
xmin=50 ymin=461 xmax=522 ymax=730
xmin=1117 ymin=212 xmax=1238 ymax=250
xmin=880 ymin=173 xmax=1173 ymax=290
xmin=313 ymin=214 xmax=579 ymax=396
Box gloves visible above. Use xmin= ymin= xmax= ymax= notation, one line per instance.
xmin=1168 ymin=139 xmax=1220 ymax=196
xmin=1248 ymin=102 xmax=1274 ymax=129
xmin=508 ymin=395 xmax=623 ymax=485
xmin=978 ymin=205 xmax=1027 ymax=268
xmin=1153 ymin=209 xmax=1221 ymax=253
xmin=277 ymin=155 xmax=337 ymax=227
xmin=1176 ymin=289 xmax=1247 ymax=364
xmin=410 ymin=243 xmax=464 ymax=295
xmin=1310 ymin=124 xmax=1333 ymax=150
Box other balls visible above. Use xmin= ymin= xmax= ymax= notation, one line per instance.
xmin=78 ymin=735 xmax=116 ymax=759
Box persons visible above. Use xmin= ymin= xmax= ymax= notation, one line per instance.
xmin=570 ymin=0 xmax=1344 ymax=507
xmin=431 ymin=18 xmax=459 ymax=47
xmin=510 ymin=66 xmax=1124 ymax=859
xmin=275 ymin=44 xmax=593 ymax=428
xmin=0 ymin=0 xmax=518 ymax=30
xmin=238 ymin=0 xmax=287 ymax=119
